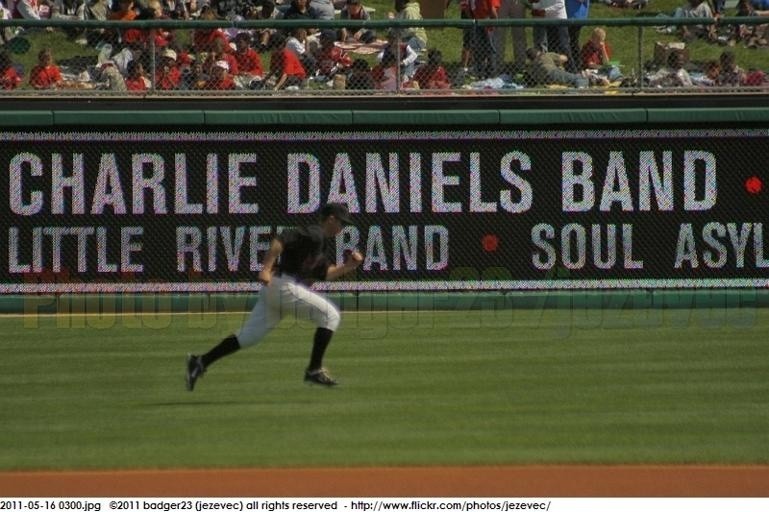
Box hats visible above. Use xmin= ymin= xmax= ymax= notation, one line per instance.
xmin=589 ymin=28 xmax=607 ymax=46
xmin=39 ymin=48 xmax=52 ymax=57
xmin=385 ymin=29 xmax=397 ymax=39
xmin=214 ymin=61 xmax=228 ymax=69
xmin=101 ymin=60 xmax=113 ymax=70
xmin=318 ymin=204 xmax=354 ymax=224
xmin=162 ymin=49 xmax=176 ymax=62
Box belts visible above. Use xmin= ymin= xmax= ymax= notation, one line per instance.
xmin=273 ymin=272 xmax=282 ymax=277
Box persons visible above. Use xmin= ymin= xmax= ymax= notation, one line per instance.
xmin=184 ymin=202 xmax=364 ymax=392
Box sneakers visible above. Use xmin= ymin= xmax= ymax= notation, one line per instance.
xmin=304 ymin=368 xmax=341 ymax=385
xmin=187 ymin=353 xmax=201 ymax=392
